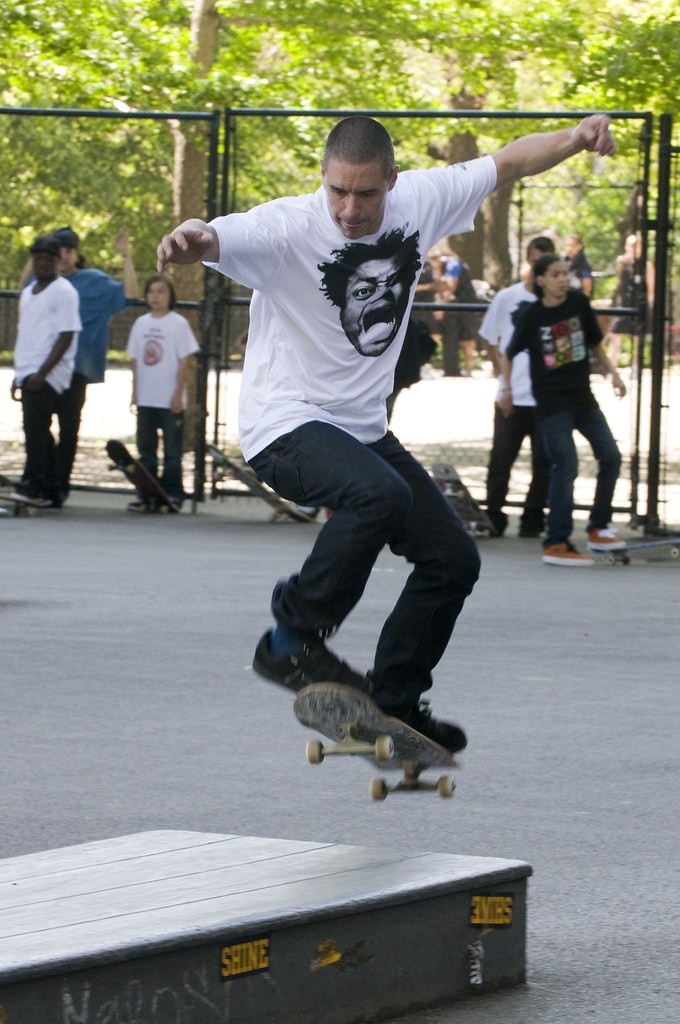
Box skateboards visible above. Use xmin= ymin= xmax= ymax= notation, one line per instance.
xmin=294 ymin=678 xmax=458 ymax=801
xmin=589 ymin=536 xmax=680 ymax=566
xmin=106 ymin=438 xmax=178 ymax=513
xmin=428 ymin=462 xmax=494 ymax=536
xmin=204 ymin=444 xmax=314 ymax=523
xmin=0 ymin=474 xmax=55 ymax=515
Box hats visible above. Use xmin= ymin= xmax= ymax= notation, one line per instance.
xmin=29 ymin=236 xmax=64 ymax=258
xmin=55 ymin=227 xmax=80 ymax=249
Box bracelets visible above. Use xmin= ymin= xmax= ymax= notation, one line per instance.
xmin=501 ymin=384 xmax=512 ymax=391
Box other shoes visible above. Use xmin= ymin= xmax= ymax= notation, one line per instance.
xmin=128 ymin=500 xmax=149 ymax=511
xmin=10 ymin=484 xmax=63 ymax=509
xmin=487 ymin=509 xmax=507 ymax=538
xmin=160 ymin=503 xmax=179 ymax=514
xmin=518 ymin=522 xmax=542 ymax=537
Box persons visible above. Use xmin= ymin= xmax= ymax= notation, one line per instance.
xmin=476 ymin=237 xmax=578 ymax=538
xmin=417 ymin=236 xmax=655 ymax=383
xmin=18 ymin=227 xmax=145 ymax=504
xmin=122 ymin=274 xmax=202 ymax=517
xmin=315 ymin=223 xmax=421 ymax=357
xmin=156 ymin=112 xmax=617 ymax=753
xmin=6 ymin=235 xmax=82 ymax=507
xmin=499 ymin=256 xmax=627 ymax=567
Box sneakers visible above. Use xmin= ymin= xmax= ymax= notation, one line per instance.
xmin=542 ymin=543 xmax=594 ymax=566
xmin=253 ymin=631 xmax=368 ymax=694
xmin=385 ymin=702 xmax=467 ymax=752
xmin=587 ymin=529 xmax=626 ymax=549
